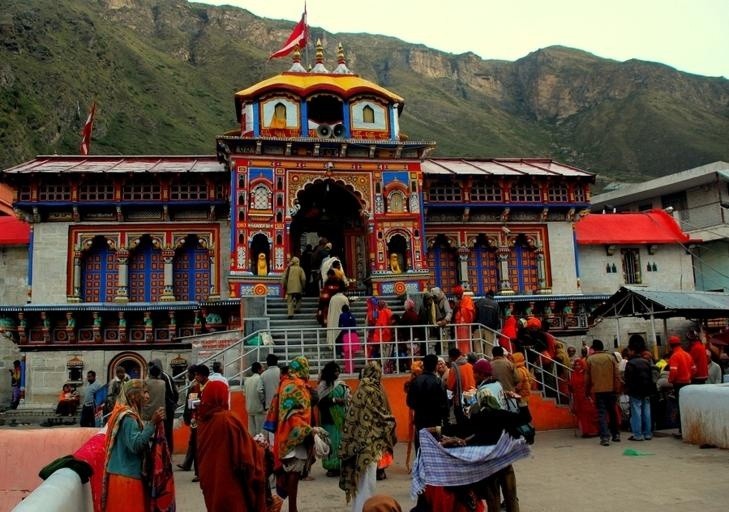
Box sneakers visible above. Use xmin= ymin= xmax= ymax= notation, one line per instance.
xmin=627 ymin=436 xmax=642 ymax=442
xmin=192 ymin=476 xmax=200 ymax=482
xmin=176 ymin=464 xmax=191 ymax=472
xmin=601 ymin=438 xmax=609 ymax=447
xmin=611 ymin=433 xmax=621 ymax=442
xmin=645 ymin=434 xmax=653 ymax=441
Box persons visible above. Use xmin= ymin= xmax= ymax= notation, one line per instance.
xmin=321 ymin=251 xmax=345 ymax=288
xmin=141 ymin=379 xmax=174 ymax=512
xmin=412 ymin=425 xmax=486 ymax=512
xmin=342 ymin=326 xmax=361 ymax=372
xmin=242 ymin=361 xmax=266 ymax=438
xmin=585 ymin=339 xmax=622 ymax=446
xmin=566 ymin=345 xmax=580 ymax=373
xmin=705 ymin=350 xmax=721 ymax=383
xmin=263 ymin=355 xmax=332 ymax=512
xmin=102 ymin=379 xmax=165 ymax=511
xmin=405 ymin=361 xmax=424 ymax=475
xmin=327 ymin=285 xmax=351 ymax=350
xmin=184 ymin=365 xmax=212 ymax=482
xmin=300 ymin=244 xmax=313 ymax=292
xmin=686 ymin=330 xmax=708 ymax=384
xmin=321 ymin=269 xmax=348 ymax=294
xmin=176 ymin=365 xmax=203 ymax=470
xmin=371 ymin=299 xmax=393 ymax=357
xmin=208 ymin=362 xmax=230 ymax=411
xmin=314 ymin=362 xmax=353 ymax=477
xmin=339 ymin=361 xmax=398 ymax=512
xmin=467 ymin=355 xmax=496 ymax=384
xmin=402 ymin=300 xmax=418 ymax=325
xmin=579 ymin=347 xmax=590 ymax=371
xmin=668 ymin=336 xmax=697 ymax=434
xmin=541 ymin=320 xmax=558 ymax=398
xmin=79 ymin=370 xmax=101 ymax=427
xmin=7 ymin=360 xmax=22 ymax=410
xmin=472 ymin=358 xmax=519 ymax=512
xmin=447 ymin=287 xmax=476 ymax=355
xmin=108 ymin=366 xmax=129 ymax=420
xmin=642 ymin=351 xmax=663 ymax=432
xmin=405 ymin=354 xmax=450 ymax=457
xmin=473 ymin=290 xmax=501 ymax=358
xmin=555 ymin=341 xmax=570 ymax=402
xmin=195 ymin=381 xmax=258 ymax=512
xmin=510 ymin=319 xmax=535 ymax=370
xmin=445 ymin=348 xmax=475 ymax=437
xmin=624 ymin=343 xmax=653 ymax=442
xmin=419 ymin=292 xmax=433 ymax=324
xmin=316 ymin=238 xmax=327 ymax=251
xmin=282 ymin=256 xmax=306 ymax=319
xmin=384 ymin=314 xmax=408 ymax=355
xmin=261 ymin=354 xmax=283 ymax=447
xmin=428 ymin=287 xmax=453 ymax=325
xmin=147 ymin=359 xmax=179 ymax=460
xmin=336 ymin=304 xmax=358 ymax=356
xmin=56 ymin=385 xmax=79 ymax=417
xmin=327 ymin=260 xmax=350 ymax=287
xmin=317 ymin=242 xmax=336 ymax=270
xmin=510 ymin=352 xmax=536 ymax=444
xmin=489 ymin=346 xmax=519 ymax=392
xmin=611 ymin=351 xmax=629 ymax=431
xmin=436 ymin=355 xmax=450 ymax=380
xmin=718 ymin=353 xmax=729 ymax=383
xmin=569 ymin=359 xmax=602 ymax=438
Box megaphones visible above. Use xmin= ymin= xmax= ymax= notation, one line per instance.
xmin=333 ymin=124 xmax=346 ymax=140
xmin=316 ymin=123 xmax=332 ymax=139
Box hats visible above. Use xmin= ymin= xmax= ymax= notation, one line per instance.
xmin=669 ymin=336 xmax=681 ymax=344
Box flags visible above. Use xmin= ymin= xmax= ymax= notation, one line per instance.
xmin=265 ymin=11 xmax=311 ymax=63
xmin=81 ymin=102 xmax=97 ymax=155
